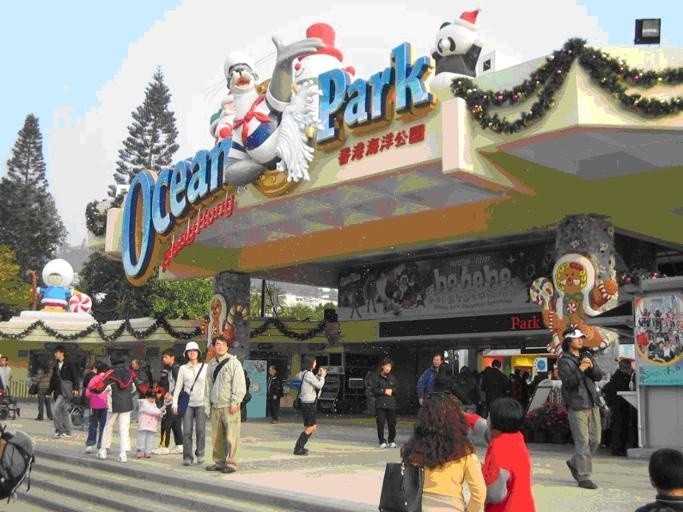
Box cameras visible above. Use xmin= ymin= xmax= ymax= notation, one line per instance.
xmin=319 ymin=369 xmax=323 ymax=374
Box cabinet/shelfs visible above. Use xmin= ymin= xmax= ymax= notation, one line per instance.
xmin=317 ymin=374 xmax=342 ymax=414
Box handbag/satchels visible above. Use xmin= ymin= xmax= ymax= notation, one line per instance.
xmin=178 ymin=390 xmax=191 ymax=414
xmin=292 ymin=396 xmax=303 ymax=410
xmin=374 ymin=448 xmax=424 ymax=512
xmin=59 ymin=381 xmax=80 ymax=400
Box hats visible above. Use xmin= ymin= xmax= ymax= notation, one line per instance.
xmin=564 ymin=328 xmax=588 ymax=341
xmin=183 ymin=340 xmax=203 ymax=358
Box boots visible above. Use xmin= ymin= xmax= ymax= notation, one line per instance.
xmin=294 ymin=432 xmax=313 ymax=455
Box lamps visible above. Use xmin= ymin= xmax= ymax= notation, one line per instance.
xmin=634 ymin=18 xmax=662 ymax=46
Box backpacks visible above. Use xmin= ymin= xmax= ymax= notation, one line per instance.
xmin=0 ymin=430 xmax=39 ymax=503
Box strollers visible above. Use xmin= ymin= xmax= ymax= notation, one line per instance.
xmin=0 ymin=385 xmax=20 ymax=420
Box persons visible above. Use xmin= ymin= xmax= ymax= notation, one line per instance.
xmin=634 ymin=448 xmax=683 ymax=512
xmin=267 ymin=365 xmax=284 ymax=424
xmin=638 ymin=308 xmax=683 ymax=361
xmin=0 ymin=335 xmax=251 ymax=473
xmin=602 ymin=360 xmax=636 ymax=455
xmin=370 ymin=358 xmax=399 ymax=449
xmin=400 ymin=326 xmax=539 ymax=512
xmin=293 ymin=354 xmax=328 ymax=455
xmin=557 ymin=326 xmax=603 ymax=489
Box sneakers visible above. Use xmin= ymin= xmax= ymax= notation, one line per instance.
xmin=379 ymin=441 xmax=397 ymax=450
xmin=203 ymin=461 xmax=237 ymax=474
xmin=84 ymin=444 xmax=94 ymax=455
xmin=51 ymin=431 xmax=72 ymax=440
xmin=135 ymin=450 xmax=152 ymax=461
xmin=180 ymin=455 xmax=205 ymax=468
xmin=567 ymin=462 xmax=599 ymax=490
xmin=150 ymin=444 xmax=185 ymax=457
xmin=116 ymin=453 xmax=128 ymax=463
xmin=34 ymin=414 xmax=44 ymax=421
xmin=95 ymin=449 xmax=108 ymax=460
